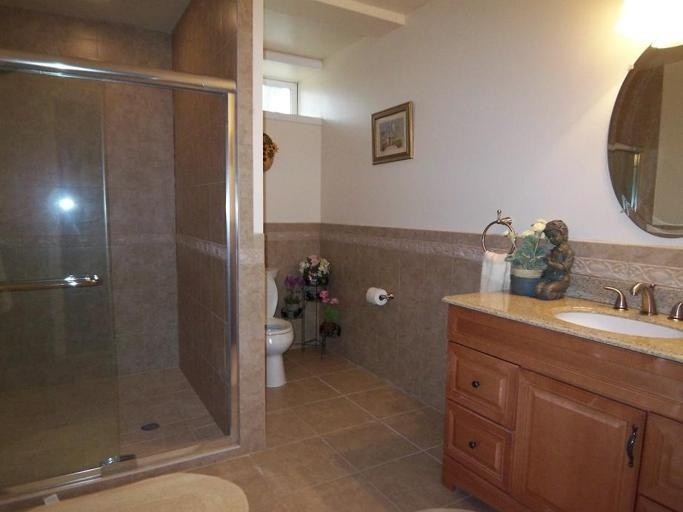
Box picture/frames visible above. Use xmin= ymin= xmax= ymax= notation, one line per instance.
xmin=371 ymin=101 xmax=413 ymax=165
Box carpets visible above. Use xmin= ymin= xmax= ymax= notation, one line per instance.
xmin=29 ymin=473 xmax=249 ymax=512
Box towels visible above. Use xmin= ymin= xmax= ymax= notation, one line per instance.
xmin=480 ymin=251 xmax=511 ymax=291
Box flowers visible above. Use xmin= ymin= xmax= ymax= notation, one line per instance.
xmin=283 ymin=275 xmax=303 ymax=304
xmin=299 ymin=255 xmax=331 ymax=286
xmin=504 ymin=219 xmax=548 ymax=269
xmin=320 ymin=291 xmax=339 ymax=322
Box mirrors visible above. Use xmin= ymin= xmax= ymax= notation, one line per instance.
xmin=607 ymin=44 xmax=683 ymax=238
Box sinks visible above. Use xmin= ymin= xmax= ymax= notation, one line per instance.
xmin=551 ymin=306 xmax=683 ymax=339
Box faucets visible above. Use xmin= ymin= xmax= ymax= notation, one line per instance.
xmin=631 ymin=281 xmax=658 ymax=316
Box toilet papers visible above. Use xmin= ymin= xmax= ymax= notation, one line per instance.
xmin=366 ymin=287 xmax=387 ymax=306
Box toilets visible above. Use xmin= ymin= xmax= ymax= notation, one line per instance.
xmin=265 ymin=268 xmax=294 ymax=388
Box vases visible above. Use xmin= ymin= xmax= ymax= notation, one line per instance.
xmin=509 ymin=266 xmax=543 ymax=296
xmin=303 ymin=285 xmax=324 ymax=299
xmin=287 ymin=302 xmax=298 ymax=311
xmin=321 ymin=320 xmax=340 ymax=337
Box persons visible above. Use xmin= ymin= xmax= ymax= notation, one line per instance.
xmin=534 ymin=221 xmax=575 ymax=300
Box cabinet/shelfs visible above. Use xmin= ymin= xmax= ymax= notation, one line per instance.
xmin=507 ymin=368 xmax=642 ymax=510
xmin=637 ymin=412 xmax=682 ymax=511
xmin=446 ymin=343 xmax=514 ymax=492
